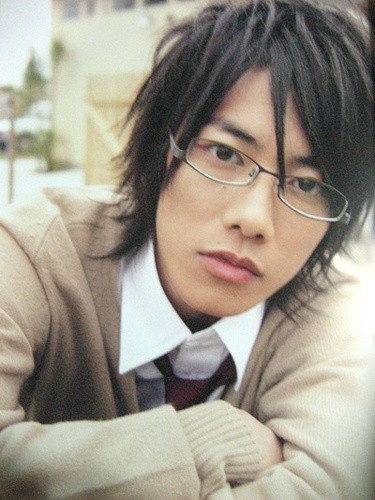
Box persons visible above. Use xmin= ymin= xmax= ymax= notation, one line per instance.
xmin=0 ymin=1 xmax=375 ymax=500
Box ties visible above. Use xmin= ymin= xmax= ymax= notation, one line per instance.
xmin=152 ymin=351 xmax=236 ymax=412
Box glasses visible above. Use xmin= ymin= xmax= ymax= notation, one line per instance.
xmin=165 ymin=128 xmax=351 ymax=228
